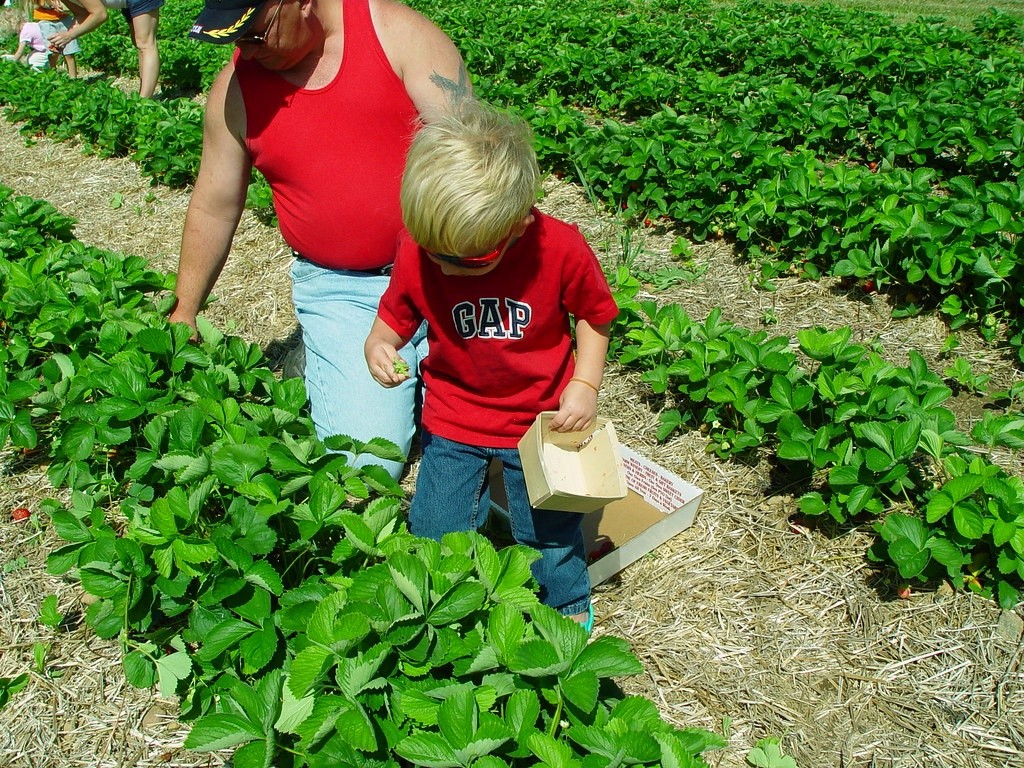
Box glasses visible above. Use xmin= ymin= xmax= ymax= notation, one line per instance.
xmin=233 ymin=0 xmax=283 ymax=47
xmin=425 ymin=227 xmax=514 ymax=269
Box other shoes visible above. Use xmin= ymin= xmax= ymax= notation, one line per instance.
xmin=573 ymin=602 xmax=594 ymax=635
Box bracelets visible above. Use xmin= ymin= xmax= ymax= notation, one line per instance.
xmin=570 ymin=378 xmax=598 ymax=395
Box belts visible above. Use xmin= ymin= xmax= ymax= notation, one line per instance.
xmin=298 ymin=252 xmax=393 ymax=276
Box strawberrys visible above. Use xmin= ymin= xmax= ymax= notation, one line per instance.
xmin=622 ymin=203 xmax=627 ymax=209
xmin=863 ymin=280 xmax=874 ymax=294
xmin=14 ymin=509 xmax=29 ymax=520
xmin=590 ymin=552 xmax=600 ymax=559
xmin=601 ymin=541 xmax=614 ymax=553
xmin=841 ymin=280 xmax=849 ymax=287
xmin=870 ymin=162 xmax=876 ymax=168
xmin=645 ymin=220 xmax=650 ymax=226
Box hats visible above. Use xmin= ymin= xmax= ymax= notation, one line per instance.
xmin=188 ymin=0 xmax=269 ymax=44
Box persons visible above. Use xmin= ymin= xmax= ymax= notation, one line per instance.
xmin=362 ymin=100 xmax=620 ymax=640
xmin=0 ymin=0 xmax=474 ymax=497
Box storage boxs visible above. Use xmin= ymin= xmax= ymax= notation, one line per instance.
xmin=581 ymin=441 xmax=706 ymax=589
xmin=515 ymin=413 xmax=629 ymax=511
